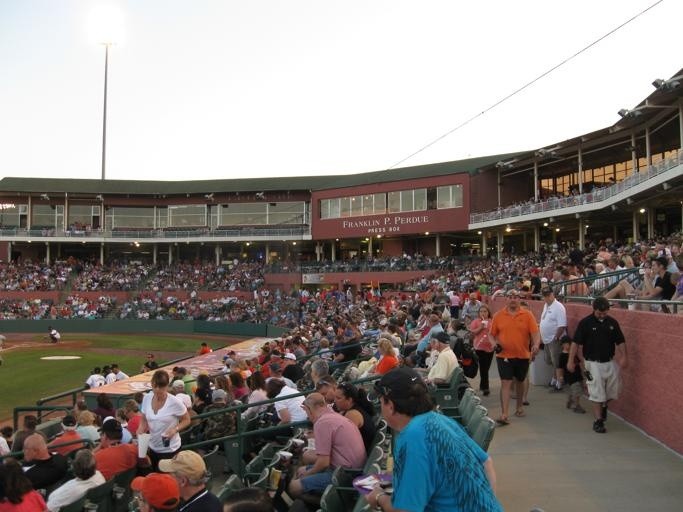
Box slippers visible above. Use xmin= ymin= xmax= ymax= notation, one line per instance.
xmin=496 ymin=416 xmax=510 ymax=425
xmin=515 ymin=410 xmax=526 ymax=417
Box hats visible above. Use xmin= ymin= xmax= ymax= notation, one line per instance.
xmin=129 ymin=472 xmax=181 ymax=509
xmin=97 ymin=419 xmax=123 ymax=431
xmin=63 ymin=415 xmax=76 ymax=426
xmin=159 ymin=449 xmax=206 ymax=478
xmin=375 ymin=368 xmax=427 ymax=399
xmin=431 ymin=331 xmax=449 ymax=345
xmin=212 ymin=388 xmax=227 ymax=401
xmin=283 ymin=353 xmax=297 ymax=360
xmin=541 ymin=286 xmax=553 ymax=295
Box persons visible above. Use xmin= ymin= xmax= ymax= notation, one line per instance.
xmin=487 ymin=288 xmax=541 ymax=424
xmin=566 ymin=297 xmax=629 ymax=434
xmin=538 ymin=286 xmax=568 ymax=394
xmin=468 ymin=176 xmax=683 ymax=315
xmin=509 ymin=299 xmax=536 ymax=406
xmin=558 ymin=336 xmax=586 ymax=414
xmin=0 ymin=249 xmax=505 ymax=511
xmin=0 ymin=221 xmax=114 ymax=241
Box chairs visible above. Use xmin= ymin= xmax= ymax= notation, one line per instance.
xmin=60 ymin=460 xmax=142 ymax=512
xmin=316 ymin=367 xmax=496 ymax=512
xmin=200 ymin=428 xmax=305 ymax=500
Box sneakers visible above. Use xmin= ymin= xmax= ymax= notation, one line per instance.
xmin=483 ymin=389 xmax=490 ymax=395
xmin=547 ymin=383 xmax=563 ymax=394
xmin=568 ymin=399 xmax=584 ymax=413
xmin=593 ymin=406 xmax=607 ymax=433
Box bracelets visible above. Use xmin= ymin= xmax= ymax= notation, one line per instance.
xmin=553 ymin=336 xmax=559 ymax=340
